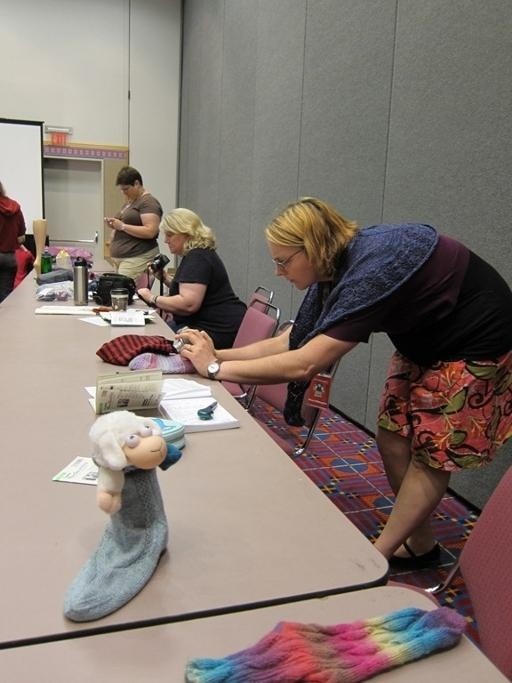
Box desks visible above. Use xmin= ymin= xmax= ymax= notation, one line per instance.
xmin=0 ymin=260 xmax=391 ymax=649
xmin=0 ymin=586 xmax=512 ymax=683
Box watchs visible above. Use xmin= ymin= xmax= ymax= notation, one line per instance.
xmin=208 ymin=356 xmax=221 ymax=378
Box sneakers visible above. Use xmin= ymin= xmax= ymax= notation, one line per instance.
xmin=389 ymin=540 xmax=442 ymax=569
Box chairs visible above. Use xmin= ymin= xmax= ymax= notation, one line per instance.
xmin=136 ymin=273 xmax=341 ymax=459
xmin=387 ymin=463 xmax=511 ymax=683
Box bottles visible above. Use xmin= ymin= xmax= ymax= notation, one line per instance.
xmin=73 ymin=255 xmax=90 ymax=306
xmin=41 ymin=248 xmax=52 ymax=274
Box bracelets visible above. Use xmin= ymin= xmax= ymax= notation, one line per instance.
xmin=121 ymin=222 xmax=126 ymax=231
xmin=148 ymin=293 xmax=159 ymax=308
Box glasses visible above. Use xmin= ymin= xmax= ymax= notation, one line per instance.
xmin=271 ymin=247 xmax=303 ymax=271
xmin=120 ymin=182 xmax=136 ymax=193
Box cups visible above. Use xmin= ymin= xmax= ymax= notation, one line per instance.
xmin=110 ymin=287 xmax=129 ymax=312
xmin=57 ymin=255 xmax=70 ymax=269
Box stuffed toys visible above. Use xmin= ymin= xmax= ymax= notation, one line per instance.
xmin=64 ymin=410 xmax=169 ymax=621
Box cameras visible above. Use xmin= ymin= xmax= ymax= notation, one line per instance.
xmin=172 ymin=326 xmax=190 ymax=354
xmin=150 ymin=254 xmax=170 ymax=272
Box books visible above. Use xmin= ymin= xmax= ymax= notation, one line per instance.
xmin=84 ymin=368 xmax=241 ymax=432
xmin=36 ymin=305 xmax=155 ymax=326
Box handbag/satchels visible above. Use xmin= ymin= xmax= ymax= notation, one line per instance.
xmin=93 ymin=273 xmax=136 ymax=307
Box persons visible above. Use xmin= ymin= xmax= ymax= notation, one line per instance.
xmin=11 ymin=236 xmax=36 ymax=291
xmin=103 ymin=166 xmax=163 ymax=287
xmin=1 ymin=182 xmax=26 ymax=304
xmin=137 ymin=206 xmax=244 ymax=350
xmin=173 ymin=197 xmax=511 ymax=571
